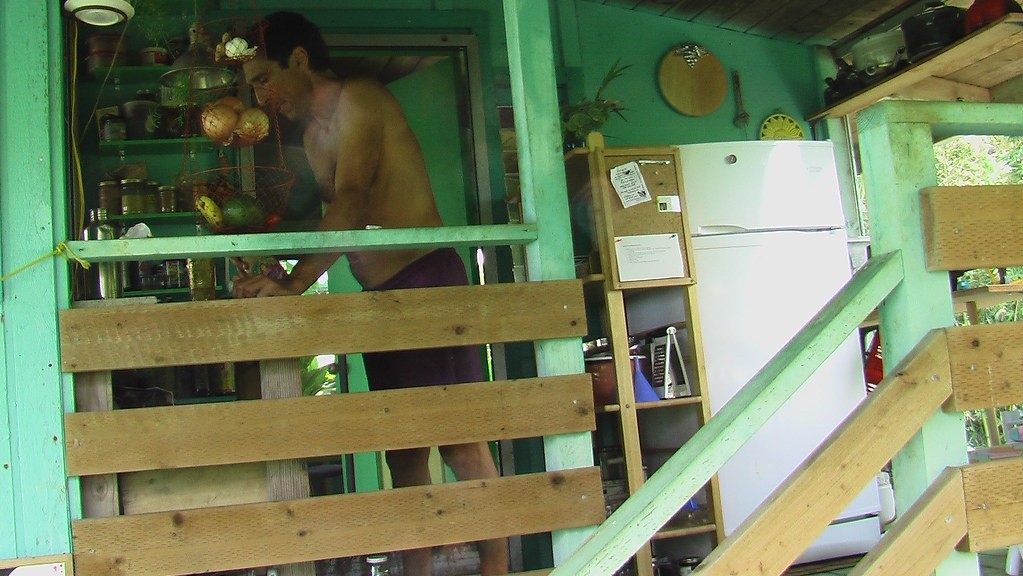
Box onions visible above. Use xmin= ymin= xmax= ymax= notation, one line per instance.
xmin=200 ymin=94 xmax=272 ymax=145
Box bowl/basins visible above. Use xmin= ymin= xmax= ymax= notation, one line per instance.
xmin=585 ymin=354 xmax=647 ymax=404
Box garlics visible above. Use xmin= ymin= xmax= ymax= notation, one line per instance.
xmin=225 ymin=36 xmax=248 ymax=59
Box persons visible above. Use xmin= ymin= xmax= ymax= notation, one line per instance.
xmin=230 ymin=11 xmax=510 ymax=575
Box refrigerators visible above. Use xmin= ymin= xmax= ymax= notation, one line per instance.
xmin=674 ymin=138 xmax=881 ymax=566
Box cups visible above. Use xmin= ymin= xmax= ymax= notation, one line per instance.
xmin=242 ymin=255 xmax=289 ymax=281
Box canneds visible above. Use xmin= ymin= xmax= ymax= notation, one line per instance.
xmin=163 ymin=258 xmax=187 ymax=289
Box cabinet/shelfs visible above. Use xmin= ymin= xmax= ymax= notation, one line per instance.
xmin=100 ymin=63 xmax=240 ymax=405
xmin=564 ymin=133 xmax=723 ymax=576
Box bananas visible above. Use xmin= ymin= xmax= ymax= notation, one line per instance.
xmin=195 ymin=195 xmax=224 ymax=229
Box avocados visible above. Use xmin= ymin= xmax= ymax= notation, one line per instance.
xmin=221 ymin=195 xmax=267 ymax=227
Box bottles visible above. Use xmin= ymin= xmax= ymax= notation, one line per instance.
xmin=214 ymin=362 xmax=237 ymax=396
xmin=191 ymin=365 xmax=212 ymax=397
xmin=83 ymin=207 xmax=123 ymax=300
